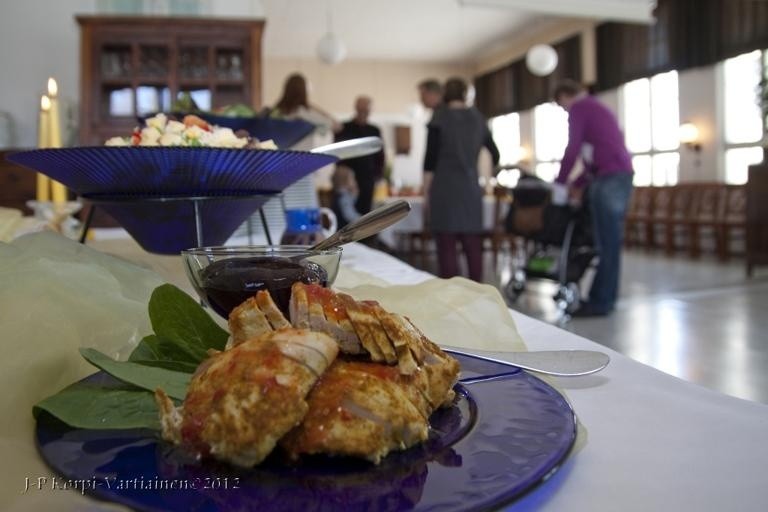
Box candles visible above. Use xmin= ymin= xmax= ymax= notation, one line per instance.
xmin=36 ymin=93 xmax=51 ymax=203
xmin=46 ymin=76 xmax=67 ymax=203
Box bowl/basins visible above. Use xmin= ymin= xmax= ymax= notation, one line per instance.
xmin=5 ymin=147 xmax=340 ymax=256
xmin=180 ymin=246 xmax=343 ymax=321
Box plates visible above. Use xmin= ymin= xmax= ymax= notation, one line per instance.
xmin=35 ymin=349 xmax=576 ymax=511
xmin=137 ymin=111 xmax=316 ymax=151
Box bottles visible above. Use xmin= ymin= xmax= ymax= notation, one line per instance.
xmin=280 ymin=208 xmax=325 ymax=245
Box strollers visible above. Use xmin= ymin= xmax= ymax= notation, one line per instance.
xmin=487 ymin=162 xmax=601 ymax=311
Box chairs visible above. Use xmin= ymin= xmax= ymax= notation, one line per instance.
xmin=624 ymin=181 xmax=752 ymax=263
xmin=368 ymin=172 xmax=553 ymax=277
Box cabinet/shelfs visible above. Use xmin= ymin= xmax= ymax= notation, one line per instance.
xmin=744 ymin=163 xmax=768 ymax=276
xmin=75 ymin=12 xmax=264 ymax=146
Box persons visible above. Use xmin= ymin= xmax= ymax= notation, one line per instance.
xmin=418 ymin=80 xmax=442 ymax=108
xmin=257 ymin=71 xmax=401 ymax=248
xmin=422 ymin=78 xmax=499 ymax=283
xmin=554 ymin=78 xmax=635 ymax=317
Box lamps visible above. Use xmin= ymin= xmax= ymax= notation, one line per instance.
xmin=676 ymin=121 xmax=705 ymax=151
xmin=524 ymin=42 xmax=560 ymax=79
xmin=315 ymin=8 xmax=347 ymax=65
